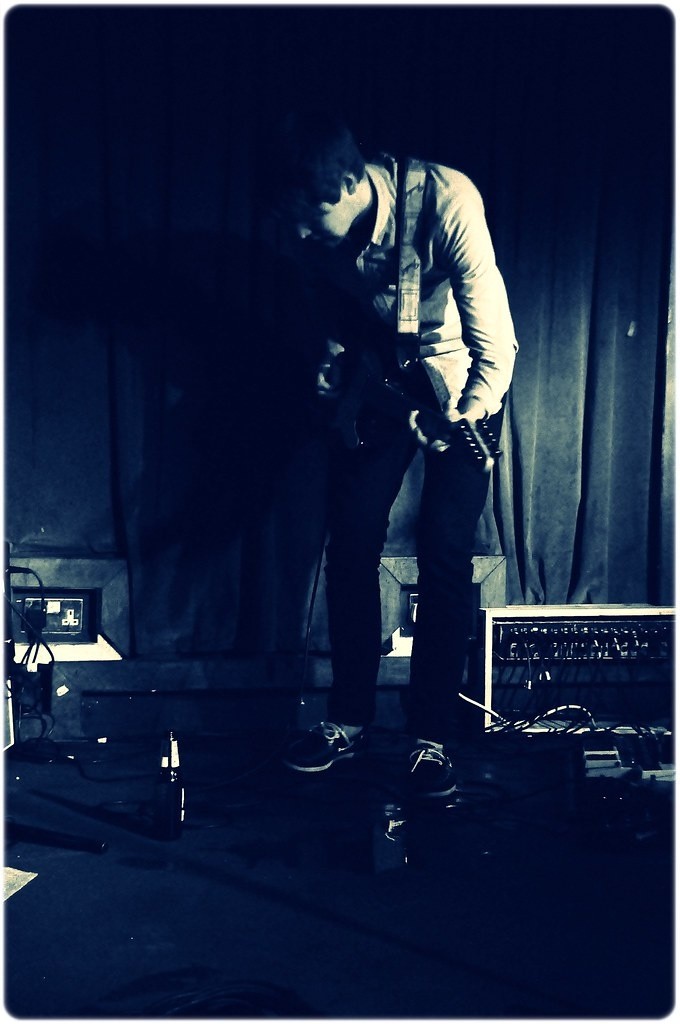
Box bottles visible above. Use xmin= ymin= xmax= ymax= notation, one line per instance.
xmin=152 ymin=732 xmax=188 ymax=838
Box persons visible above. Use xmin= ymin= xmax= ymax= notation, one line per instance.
xmin=256 ymin=121 xmax=519 ymax=799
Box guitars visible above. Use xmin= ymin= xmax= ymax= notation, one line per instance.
xmin=301 ymin=352 xmax=503 ymax=474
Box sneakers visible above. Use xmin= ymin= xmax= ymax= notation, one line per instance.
xmin=406 ymin=740 xmax=458 ymax=797
xmin=279 ymin=722 xmax=365 ymax=773
